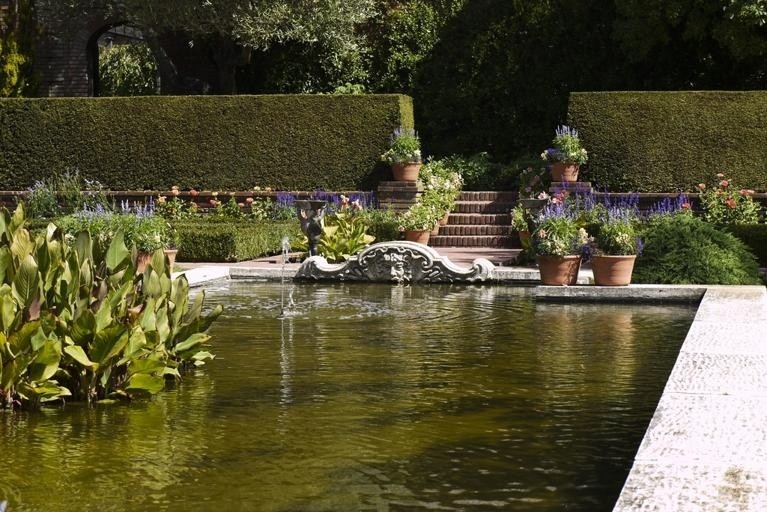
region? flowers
[135,214,177,250]
[540,124,587,162]
[397,151,492,230]
[509,166,688,257]
[378,127,422,162]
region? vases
[537,254,636,287]
[551,162,580,182]
[136,248,178,276]
[392,162,421,181]
[407,225,439,247]
